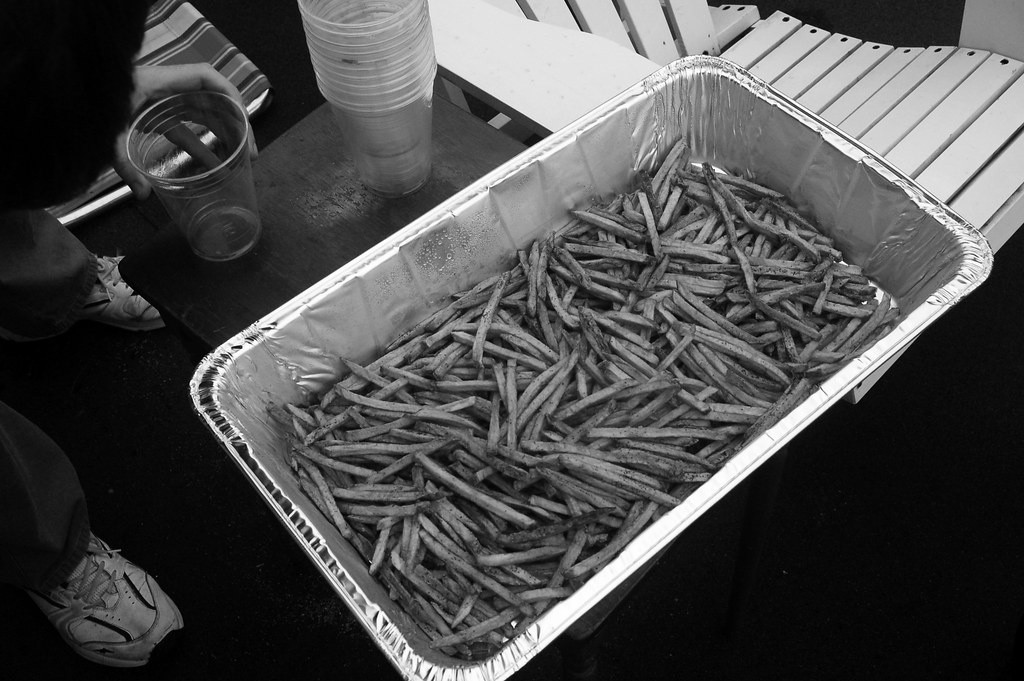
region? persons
[0,1,255,671]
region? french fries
[266,135,902,661]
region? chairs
[363,0,1024,404]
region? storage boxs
[188,56,993,681]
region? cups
[126,89,263,261]
[297,0,438,200]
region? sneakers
[20,526,184,668]
[81,255,165,330]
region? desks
[117,96,532,354]
